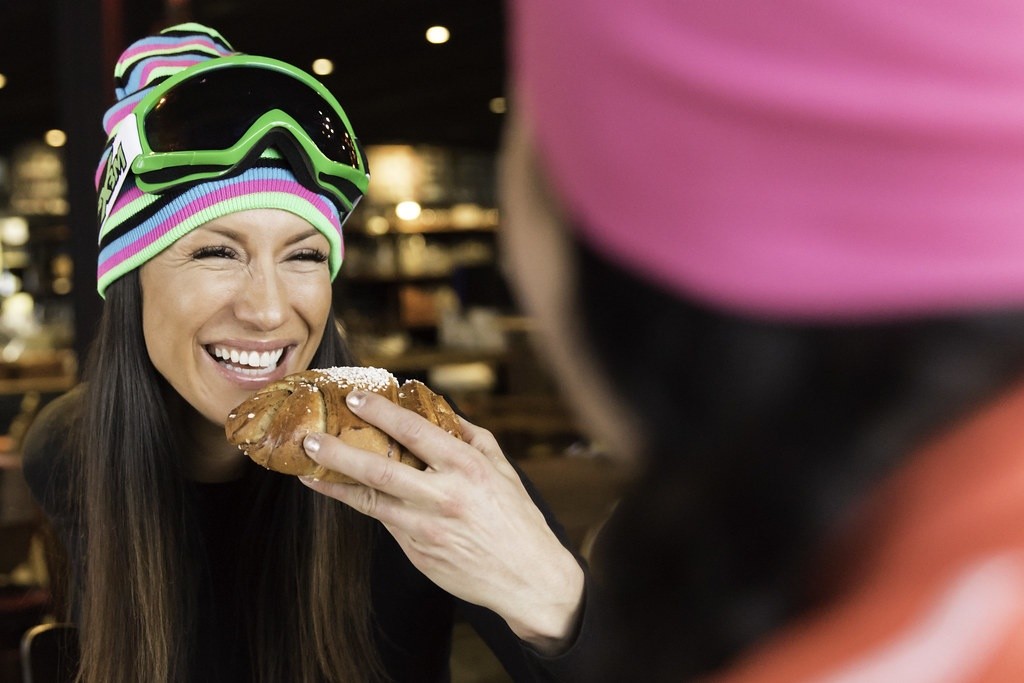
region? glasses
[96,55,370,249]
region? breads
[225,366,464,487]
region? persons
[501,0,1022,683]
[0,21,594,681]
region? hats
[512,0,1024,319]
[95,22,345,300]
